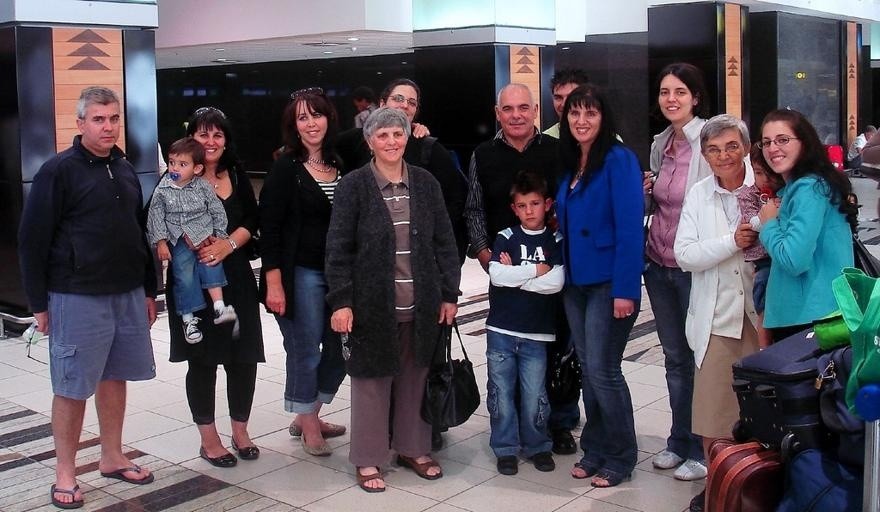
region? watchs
[227,237,237,250]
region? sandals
[572,462,596,479]
[355,466,388,492]
[592,472,622,487]
[399,453,444,480]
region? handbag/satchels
[544,339,582,397]
[853,236,880,277]
[420,359,480,431]
[704,267,879,512]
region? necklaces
[205,173,223,189]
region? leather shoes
[200,443,237,468]
[231,435,259,458]
[301,432,333,456]
[552,430,578,454]
[289,420,346,435]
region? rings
[210,255,214,260]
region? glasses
[757,137,797,151]
[290,88,324,98]
[25,325,49,368]
[193,107,226,121]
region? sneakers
[182,318,204,344]
[214,304,237,324]
[497,456,518,474]
[653,449,683,468]
[673,458,707,482]
[532,451,555,472]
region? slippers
[51,484,84,510]
[101,464,154,485]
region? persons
[143,106,260,468]
[18,86,154,510]
[847,126,879,178]
[465,70,646,487]
[149,136,238,347]
[644,66,860,481]
[261,81,467,495]
